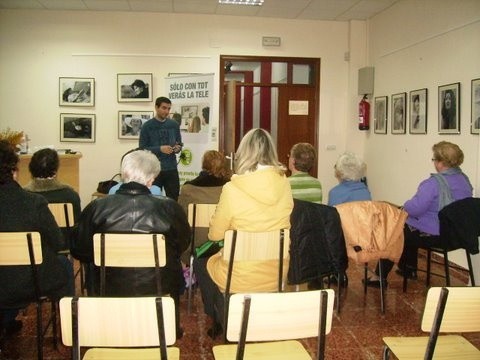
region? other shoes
[0,265,425,342]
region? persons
[69,148,194,341]
[411,95,421,129]
[19,148,83,303]
[176,150,235,294]
[139,97,184,205]
[278,141,324,206]
[201,105,210,124]
[440,88,457,130]
[0,136,63,352]
[362,140,474,289]
[189,116,202,133]
[107,147,163,198]
[320,153,372,289]
[67,88,90,104]
[122,113,145,136]
[196,128,295,338]
[131,79,149,99]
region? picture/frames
[60,111,96,142]
[409,88,427,135]
[373,96,388,134]
[437,82,460,135]
[470,78,480,135]
[117,110,154,140]
[58,76,95,107]
[391,92,407,135]
[117,72,154,103]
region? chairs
[381,286,480,360]
[212,288,336,360]
[44,203,84,297]
[58,296,180,360]
[402,196,480,293]
[326,200,394,312]
[88,232,168,297]
[219,228,292,339]
[186,202,220,320]
[0,232,62,360]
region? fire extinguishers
[358,94,370,131]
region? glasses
[431,158,439,161]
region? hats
[125,114,148,128]
[131,80,145,89]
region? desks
[17,148,84,202]
